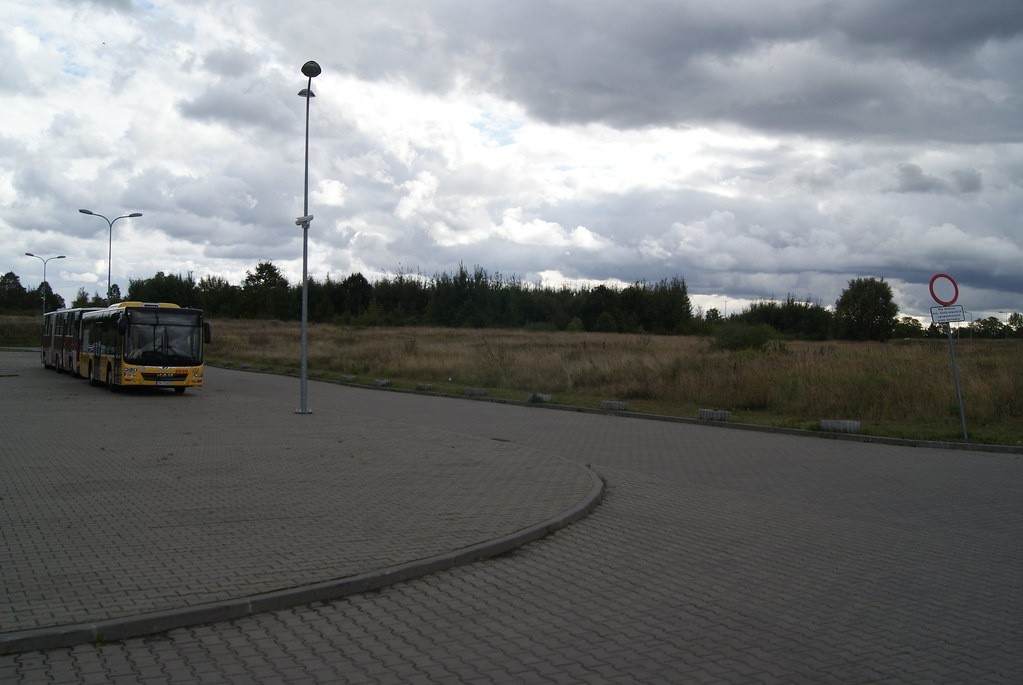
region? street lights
[24,252,66,327]
[295,60,323,414]
[79,209,143,308]
[722,299,729,319]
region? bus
[79,301,212,395]
[42,306,108,377]
